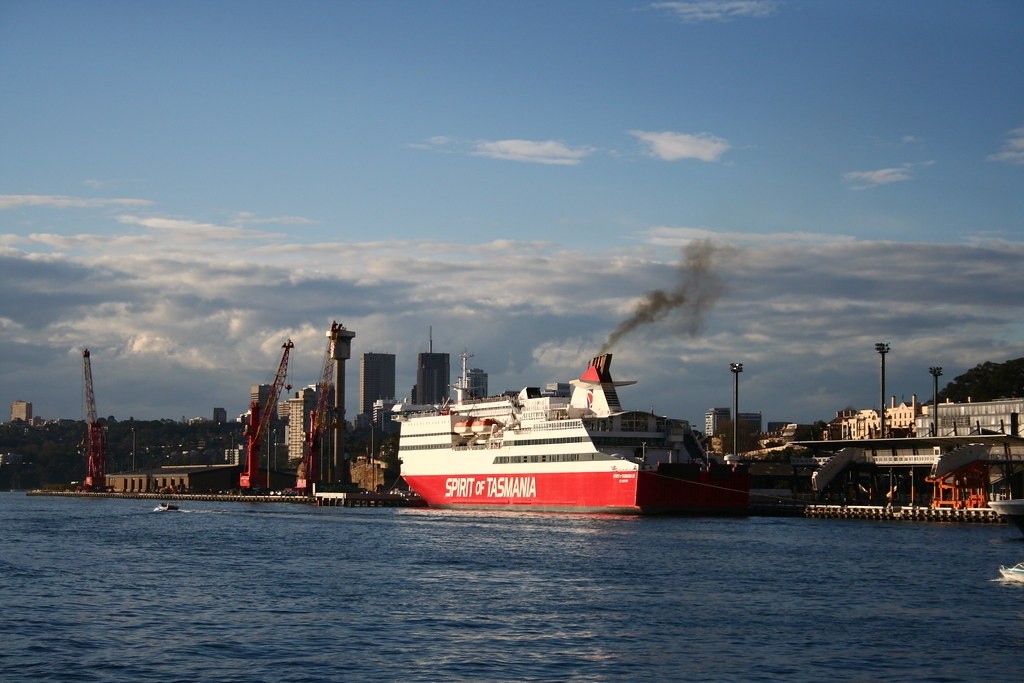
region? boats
[344,492,428,508]
[158,502,179,511]
[1000,563,1024,584]
[397,352,751,517]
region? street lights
[369,420,374,490]
[873,342,887,440]
[929,366,941,436]
[730,361,743,456]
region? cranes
[240,338,295,496]
[81,348,106,492]
[291,322,341,496]
